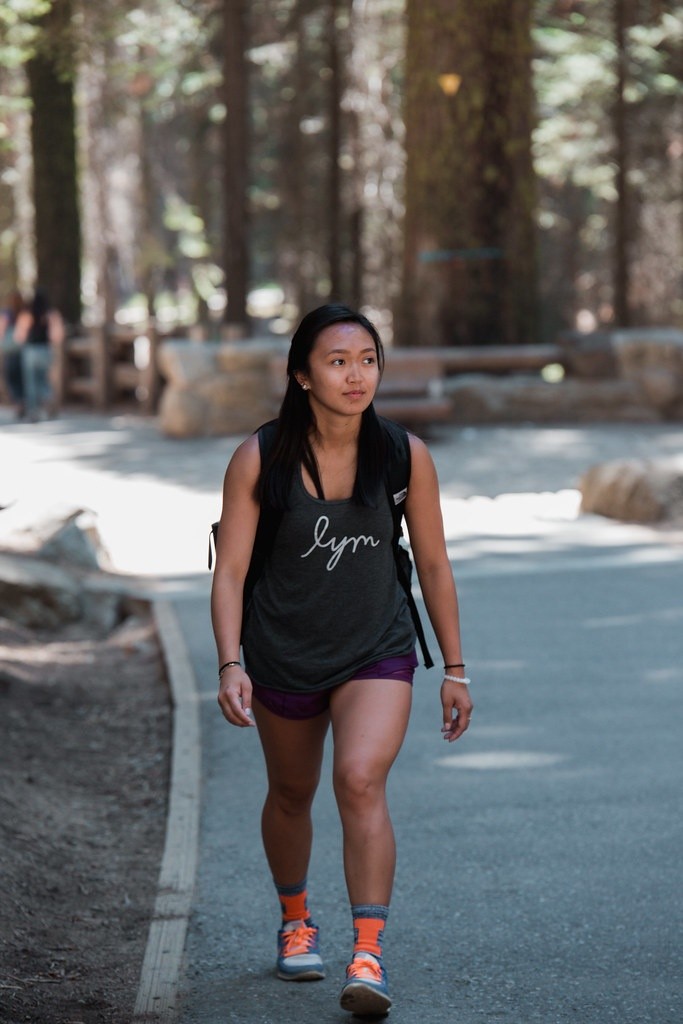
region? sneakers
[277,919,326,980]
[341,951,392,1017]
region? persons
[210,304,473,1019]
[0,285,66,423]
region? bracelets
[444,664,465,669]
[218,661,241,680]
[444,675,470,684]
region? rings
[467,714,472,720]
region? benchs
[371,398,456,440]
